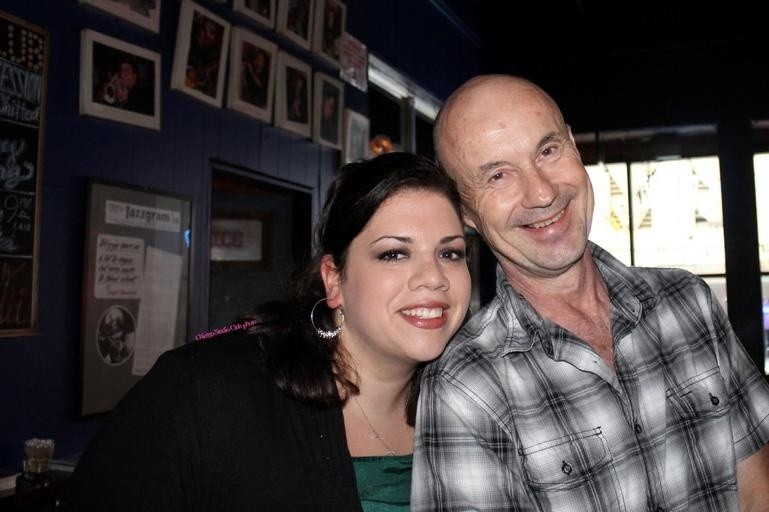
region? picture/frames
[79,176,196,417]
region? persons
[103,60,149,114]
[186,20,224,97]
[410,73,769,512]
[98,309,135,364]
[241,52,268,108]
[57,152,472,512]
[322,93,338,144]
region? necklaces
[351,392,396,456]
